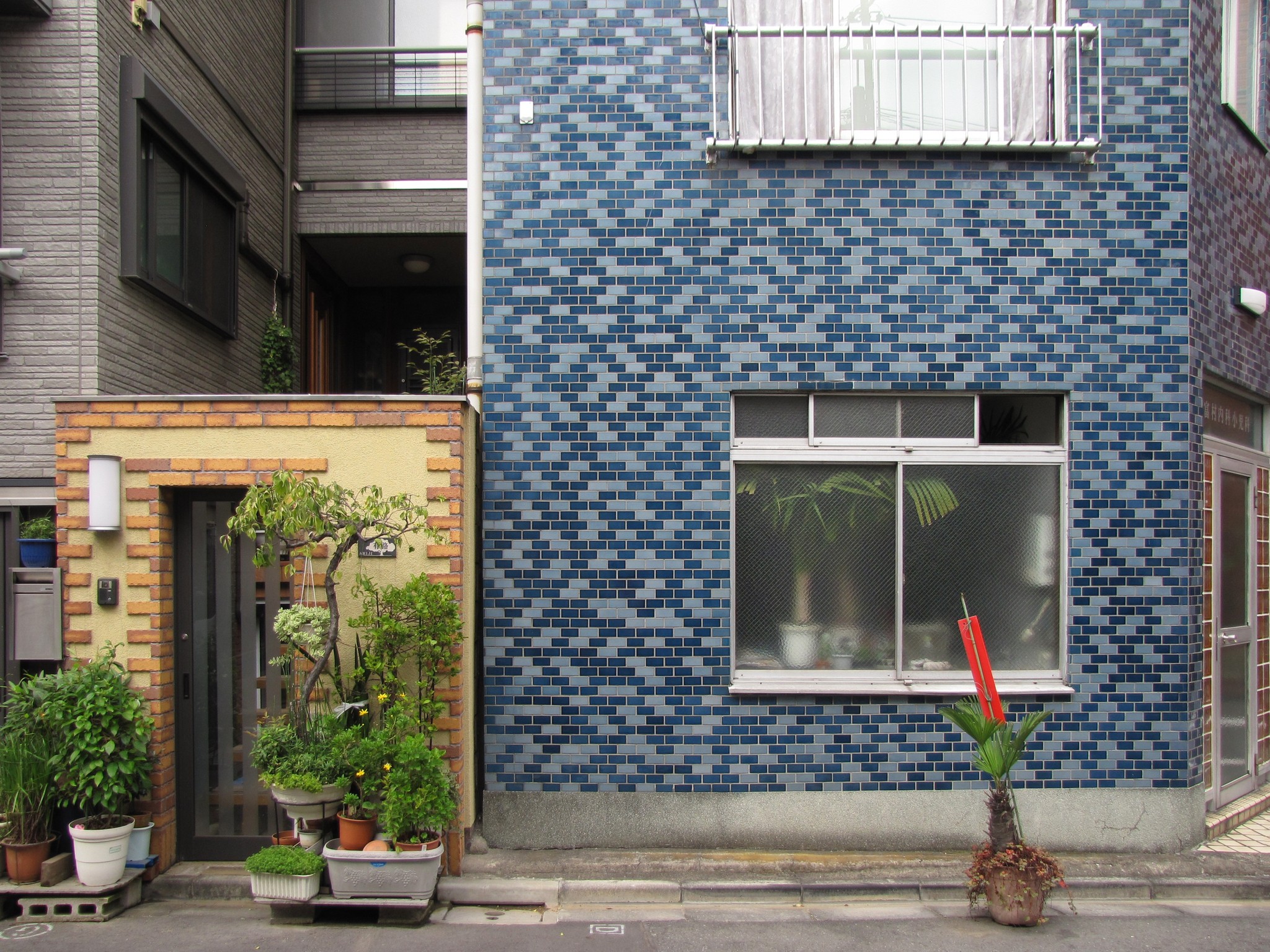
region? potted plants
[17,517,56,568]
[937,694,1081,927]
[219,471,468,901]
[0,640,155,886]
[736,463,960,670]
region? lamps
[1233,285,1266,318]
